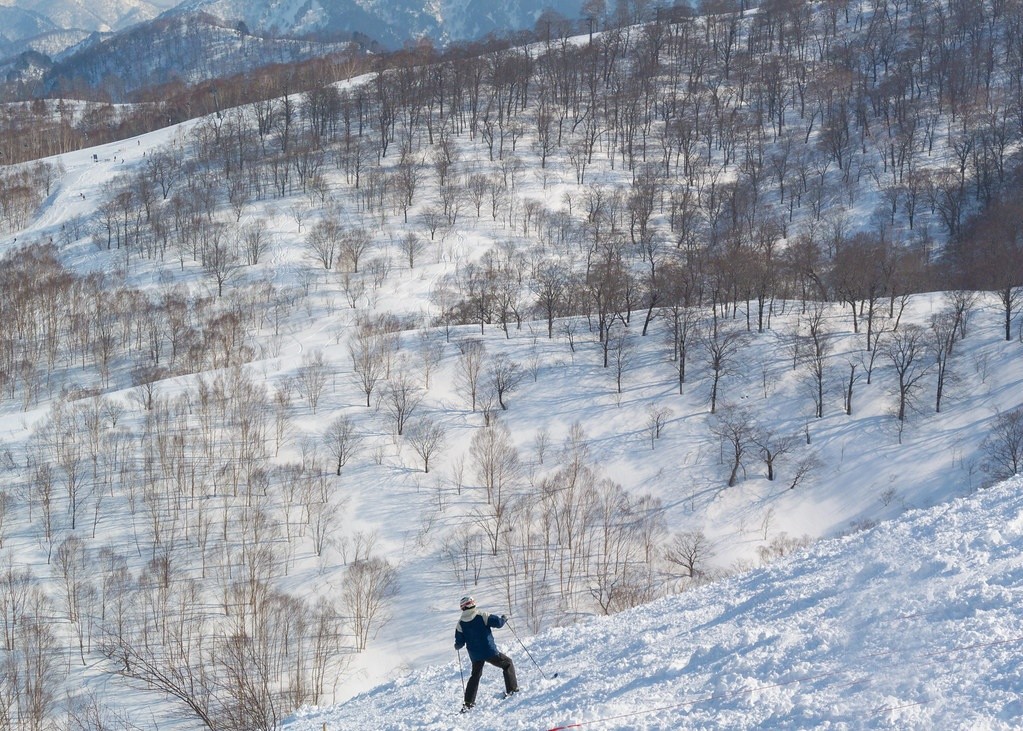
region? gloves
[501,614,509,623]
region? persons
[454,596,519,711]
[11,139,177,244]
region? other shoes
[508,688,521,694]
[466,702,476,710]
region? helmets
[460,596,475,612]
